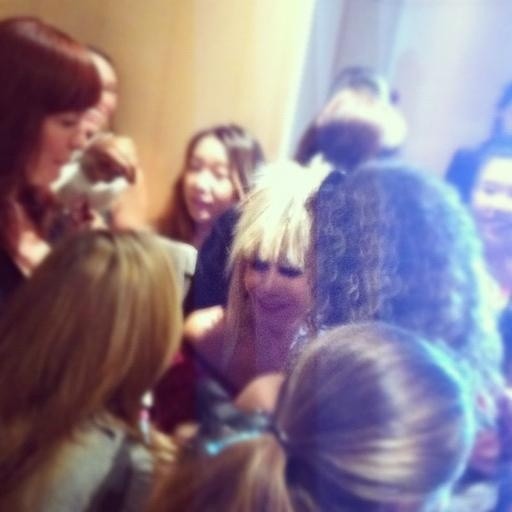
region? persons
[0,229,188,512]
[179,157,349,395]
[441,82,510,380]
[181,87,412,316]
[0,13,101,301]
[442,132,511,512]
[45,38,152,231]
[145,118,268,251]
[325,62,398,107]
[306,155,506,441]
[144,314,478,511]
